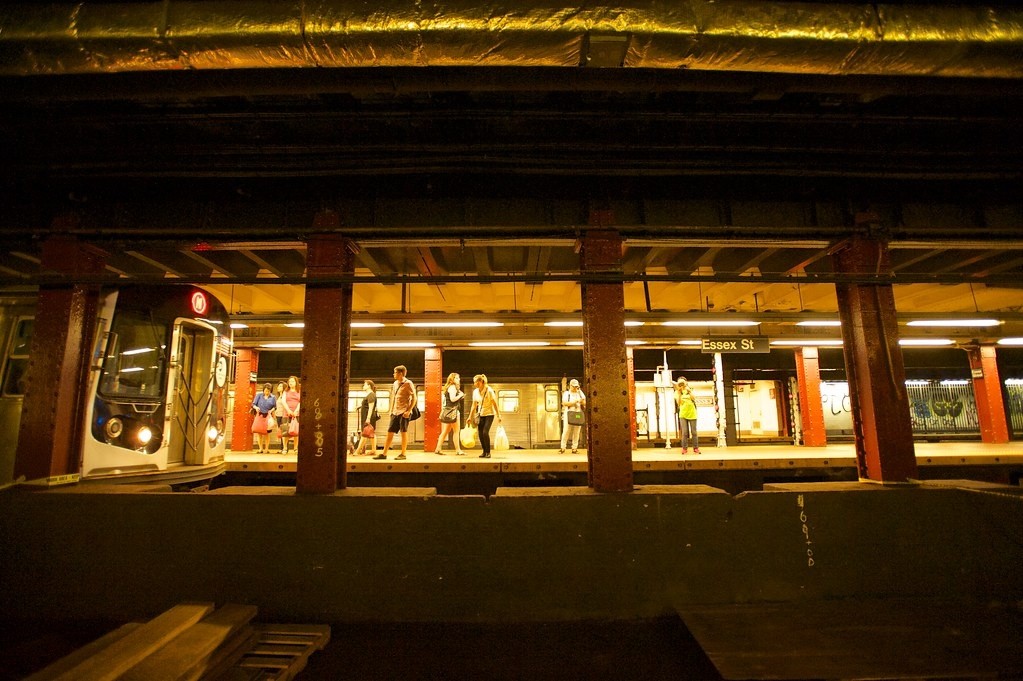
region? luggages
[350,409,366,454]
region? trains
[0,284,236,490]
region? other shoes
[435,449,446,455]
[572,449,580,454]
[456,450,468,456]
[682,447,687,454]
[558,449,566,455]
[373,454,386,459]
[353,452,360,456]
[694,447,702,454]
[365,452,376,456]
[395,454,406,460]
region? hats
[570,378,579,387]
[677,379,686,387]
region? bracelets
[584,406,585,408]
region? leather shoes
[480,453,490,458]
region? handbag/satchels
[568,411,585,426]
[494,424,509,452]
[361,423,375,438]
[278,416,299,437]
[266,410,278,430]
[410,405,421,420]
[439,406,457,423]
[471,413,480,428]
[249,408,256,416]
[366,411,380,423]
[460,423,478,448]
[251,412,268,435]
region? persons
[282,376,300,455]
[435,373,467,455]
[673,377,701,454]
[373,365,416,460]
[467,374,502,458]
[249,383,277,454]
[276,381,288,455]
[353,380,377,456]
[558,379,586,454]
